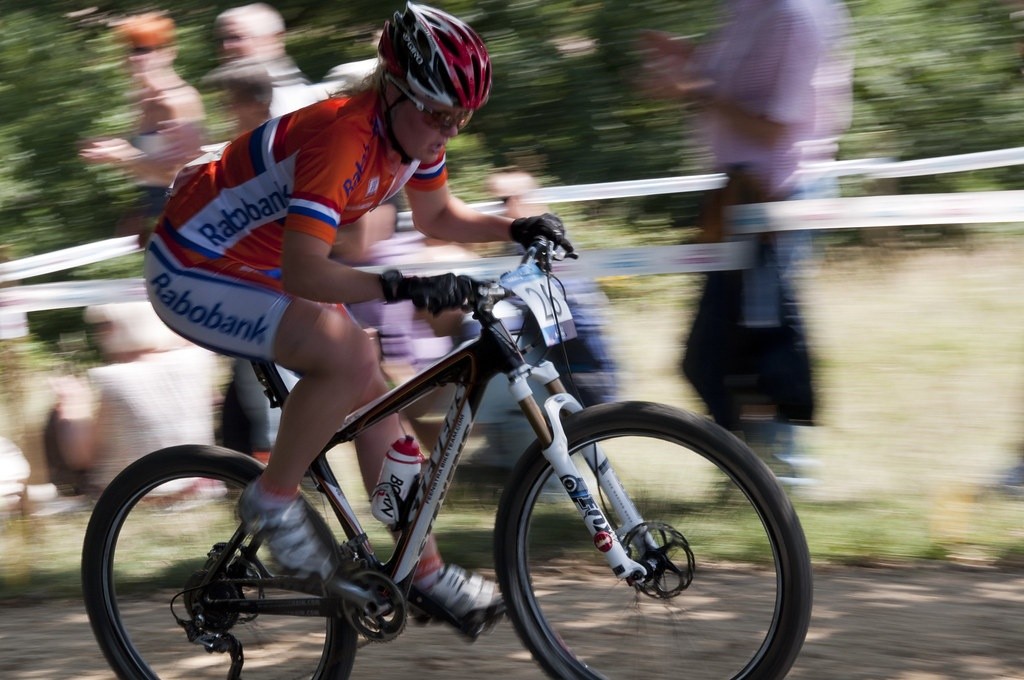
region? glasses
[379,70,475,130]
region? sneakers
[398,559,507,624]
[237,480,318,573]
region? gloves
[509,212,575,261]
[378,266,469,319]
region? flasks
[371,433,420,528]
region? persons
[136,0,572,627]
[637,0,860,502]
[2,3,621,516]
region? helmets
[374,4,496,113]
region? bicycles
[81,211,815,679]
